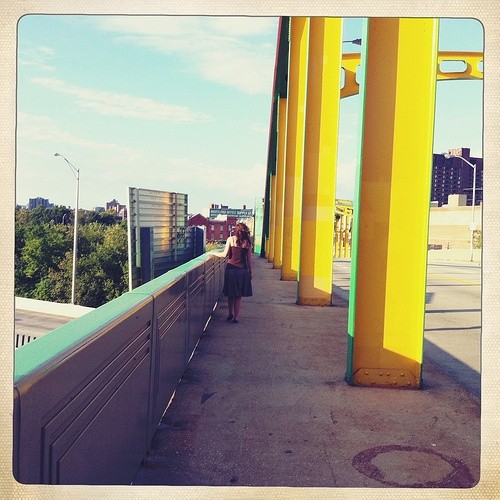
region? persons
[206,222,253,324]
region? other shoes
[232,320,239,323]
[226,314,233,321]
